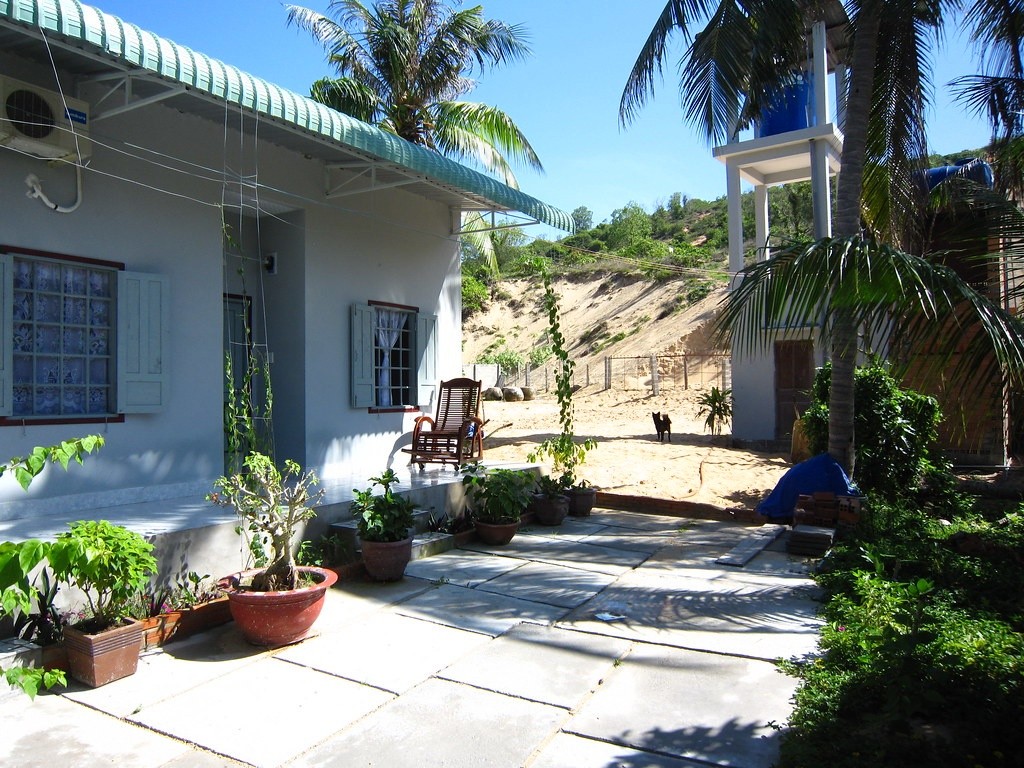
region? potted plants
[207,454,339,649]
[52,518,156,688]
[459,462,536,548]
[564,471,598,518]
[353,476,418,583]
[534,475,570,527]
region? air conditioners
[0,74,90,164]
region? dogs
[652,412,672,442]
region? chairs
[401,378,484,470]
